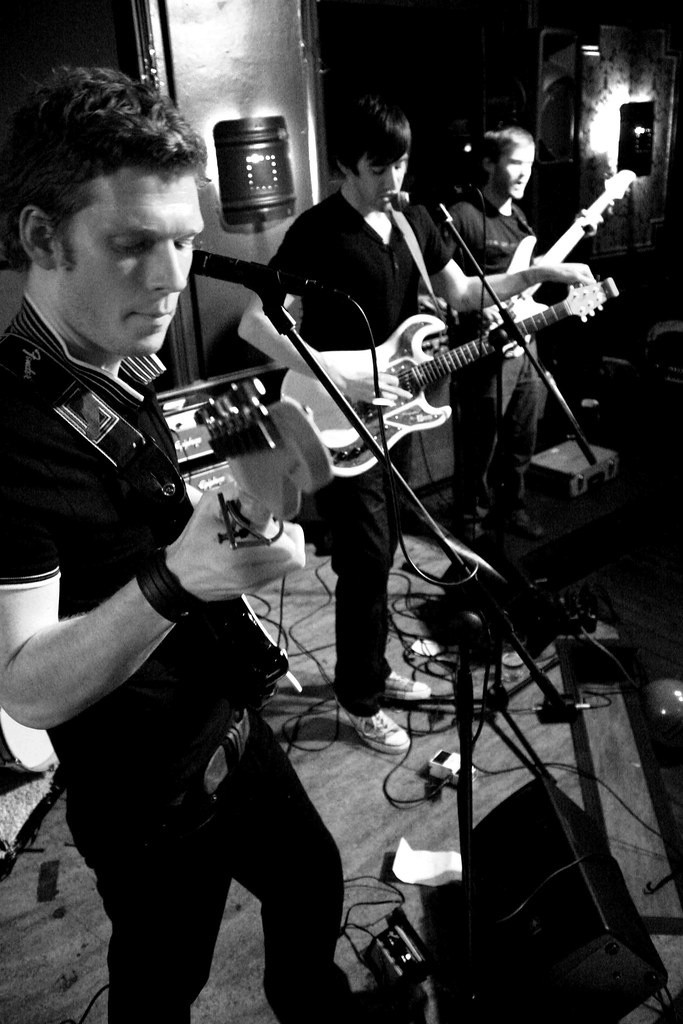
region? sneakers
[383,670,431,701]
[334,694,411,754]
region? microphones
[188,249,351,302]
[391,183,476,210]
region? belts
[170,706,250,806]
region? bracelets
[133,547,208,624]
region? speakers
[0,0,144,271]
[467,773,669,1024]
[507,26,579,166]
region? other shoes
[505,510,545,539]
[352,986,428,1024]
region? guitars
[193,376,333,538]
[279,272,621,479]
[484,170,638,359]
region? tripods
[382,207,599,783]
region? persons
[1,65,359,1024]
[238,97,599,755]
[440,124,565,542]
